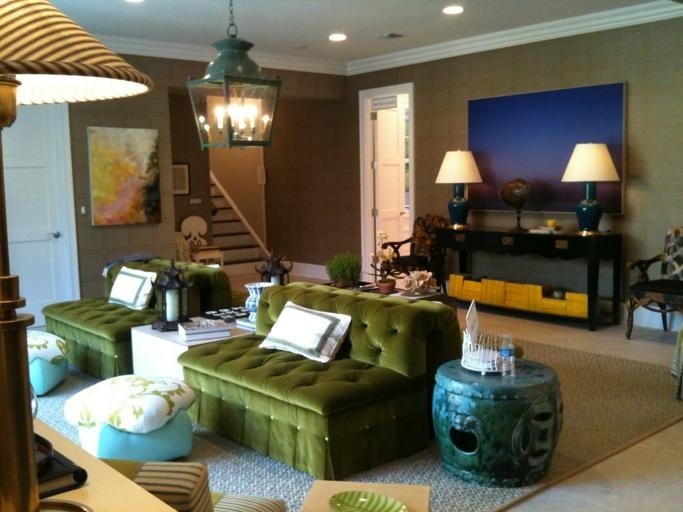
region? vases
[377,279,395,294]
[241,281,275,321]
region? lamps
[148,260,190,331]
[186,0,283,152]
[0,0,152,512]
[252,248,294,288]
[434,149,482,226]
[560,141,621,236]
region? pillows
[104,265,157,312]
[254,302,351,363]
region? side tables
[131,322,252,379]
[434,355,561,488]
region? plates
[328,489,407,511]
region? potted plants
[327,251,363,287]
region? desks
[435,224,621,334]
[321,281,457,318]
[298,479,429,512]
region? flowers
[369,247,405,281]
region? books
[34,448,86,499]
[177,318,231,342]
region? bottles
[499,334,517,379]
[243,281,275,322]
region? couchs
[26,328,66,397]
[40,257,252,380]
[62,373,194,460]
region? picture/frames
[169,161,192,196]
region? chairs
[181,214,224,266]
[98,459,289,512]
[625,229,682,337]
[384,216,448,287]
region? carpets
[0,298,682,512]
